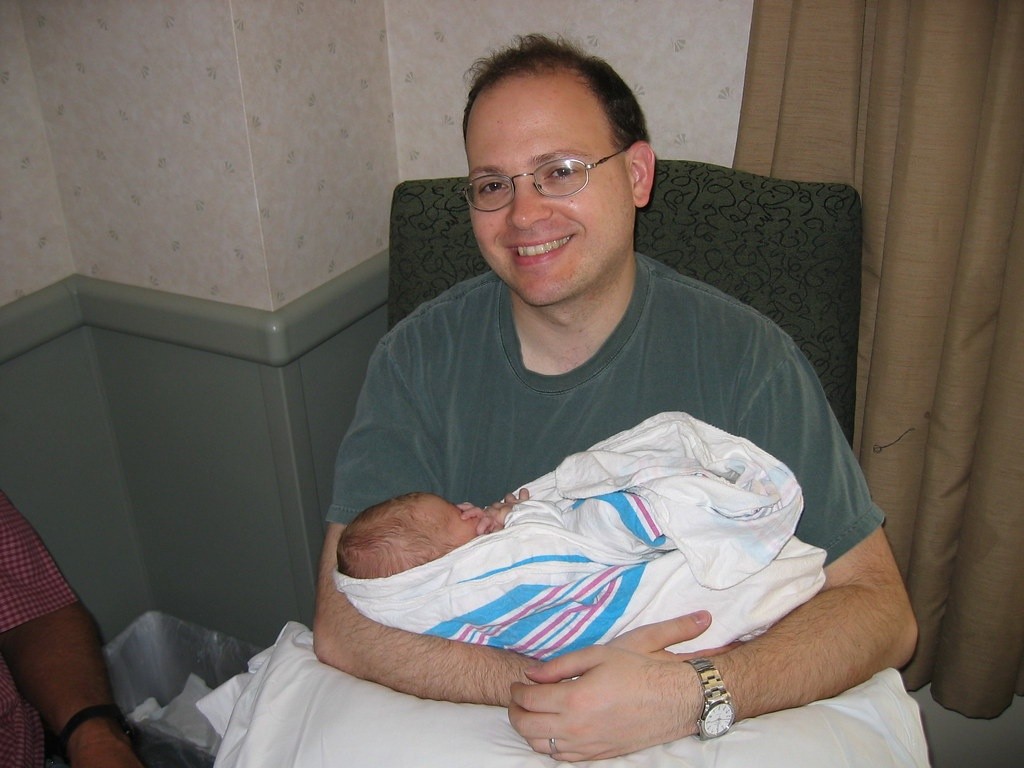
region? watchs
[684,653,733,742]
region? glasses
[459,145,629,212]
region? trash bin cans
[101,609,266,768]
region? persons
[307,37,923,767]
[1,491,141,766]
[334,489,537,580]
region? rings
[548,738,559,755]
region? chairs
[387,159,861,450]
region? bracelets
[59,704,136,760]
